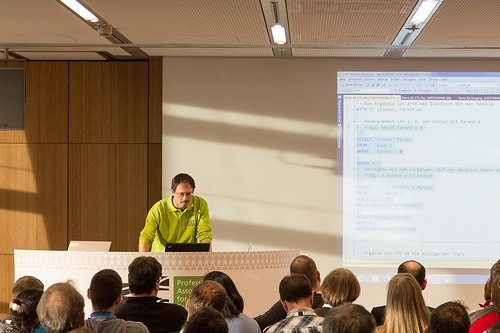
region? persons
[139,173,212,252]
[0,255,500,333]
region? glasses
[120,294,127,301]
[175,192,193,197]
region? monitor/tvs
[165,243,210,252]
